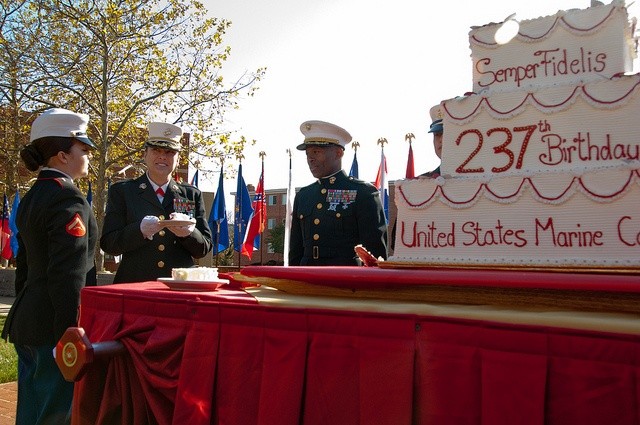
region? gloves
[139,215,165,240]
[167,218,197,238]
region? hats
[30,107,98,150]
[427,104,443,134]
[296,120,352,151]
[146,122,184,152]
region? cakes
[169,211,189,221]
[387,0,640,269]
[171,267,217,281]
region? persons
[418,103,444,177]
[0,108,99,424]
[99,121,213,284]
[286,120,388,267]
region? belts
[303,246,338,260]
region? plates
[160,219,195,228]
[156,275,229,292]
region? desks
[72,281,640,425]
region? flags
[102,174,119,273]
[85,181,93,215]
[241,170,267,261]
[190,172,202,188]
[373,151,389,227]
[1,191,11,267]
[172,170,183,182]
[402,145,418,180]
[349,153,360,178]
[231,167,255,251]
[208,168,229,257]
[284,166,294,268]
[7,187,21,262]
